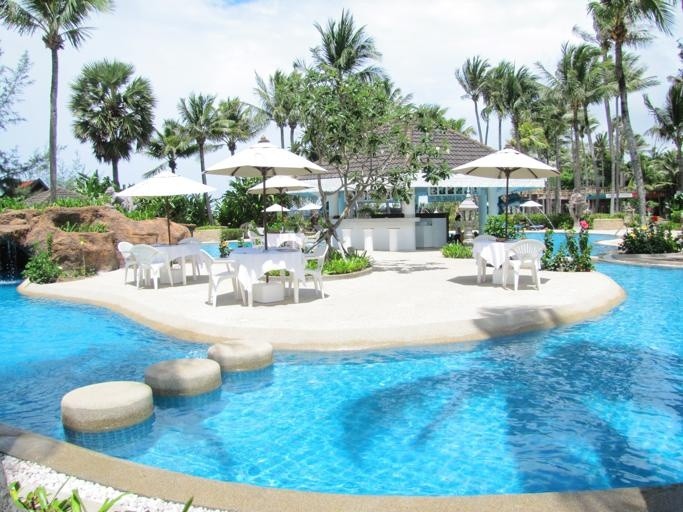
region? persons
[453,211,462,235]
[309,210,320,232]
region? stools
[362,229,375,252]
[340,228,353,250]
[387,228,399,251]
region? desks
[471,240,546,290]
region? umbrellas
[449,143,561,240]
[297,201,322,210]
[113,168,218,268]
[519,199,544,214]
[260,204,289,213]
[248,175,323,230]
[201,132,328,284]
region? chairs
[502,238,547,291]
[471,233,500,285]
[116,224,332,308]
